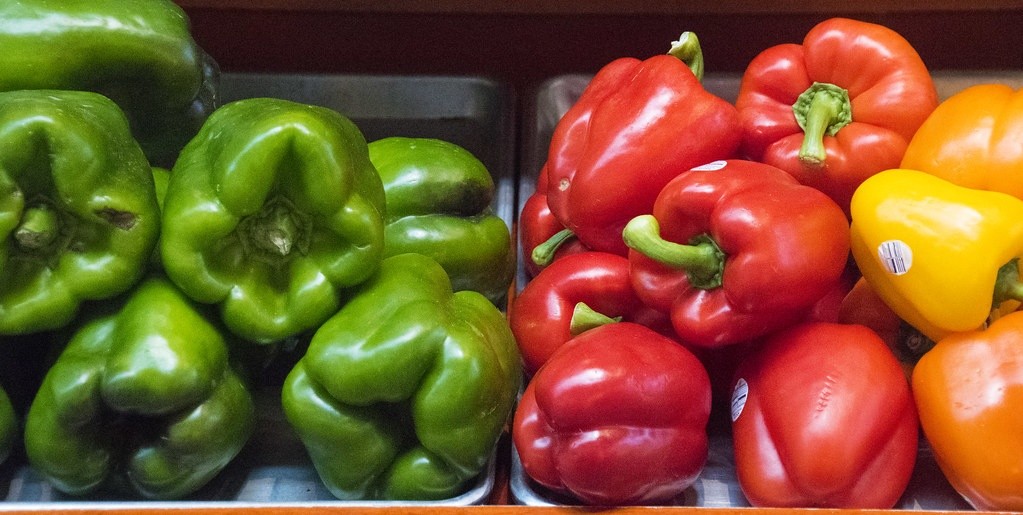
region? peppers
[0,0,1023,510]
